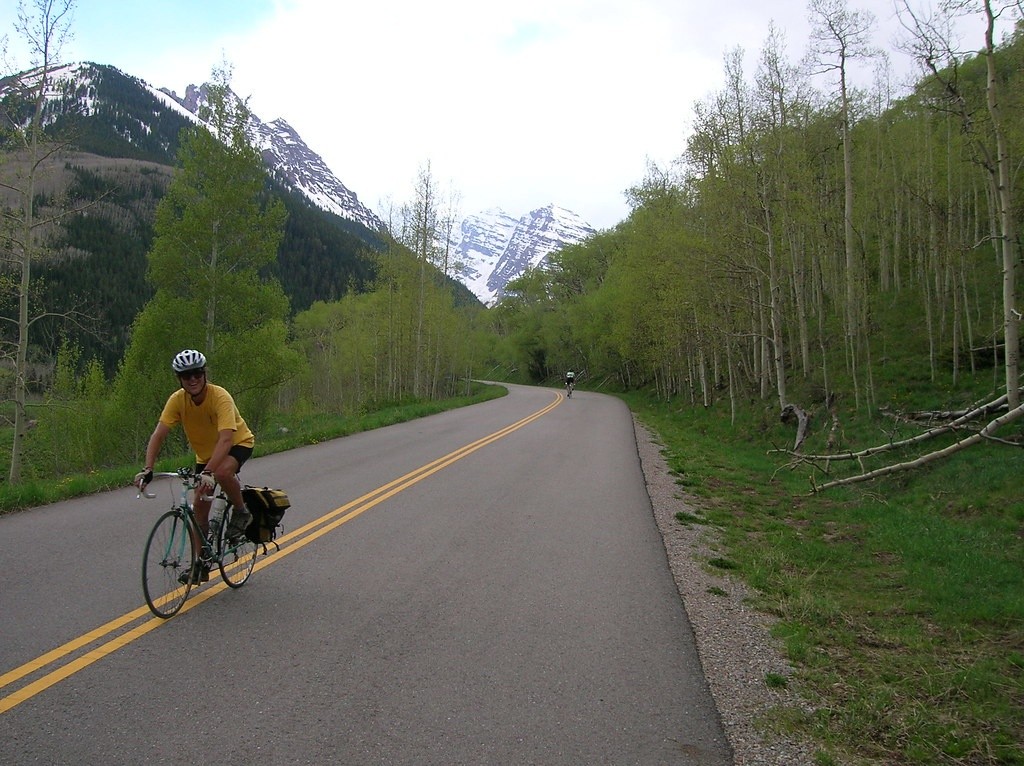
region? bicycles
[565,383,573,399]
[136,467,259,620]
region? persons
[566,368,575,397]
[133,350,254,583]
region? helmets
[172,350,208,372]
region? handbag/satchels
[242,485,291,555]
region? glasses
[179,369,204,379]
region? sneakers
[224,509,254,540]
[182,564,210,583]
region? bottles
[209,491,226,527]
